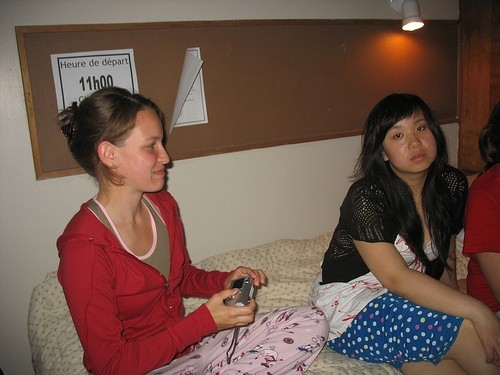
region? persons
[309,92,500,375]
[462,102,500,323]
[57,87,329,375]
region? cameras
[225,276,254,307]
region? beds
[27,228,471,375]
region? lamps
[390,0,424,31]
